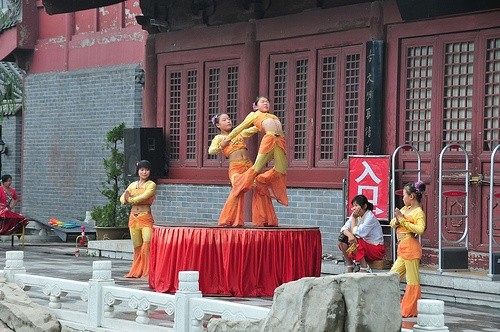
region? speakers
[123,126,164,180]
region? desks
[148,222,322,297]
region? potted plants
[90,122,131,240]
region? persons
[209,113,260,227]
[120,159,157,279]
[337,195,385,273]
[0,175,28,235]
[389,181,426,317]
[220,96,289,207]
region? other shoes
[360,264,373,273]
[347,262,356,269]
